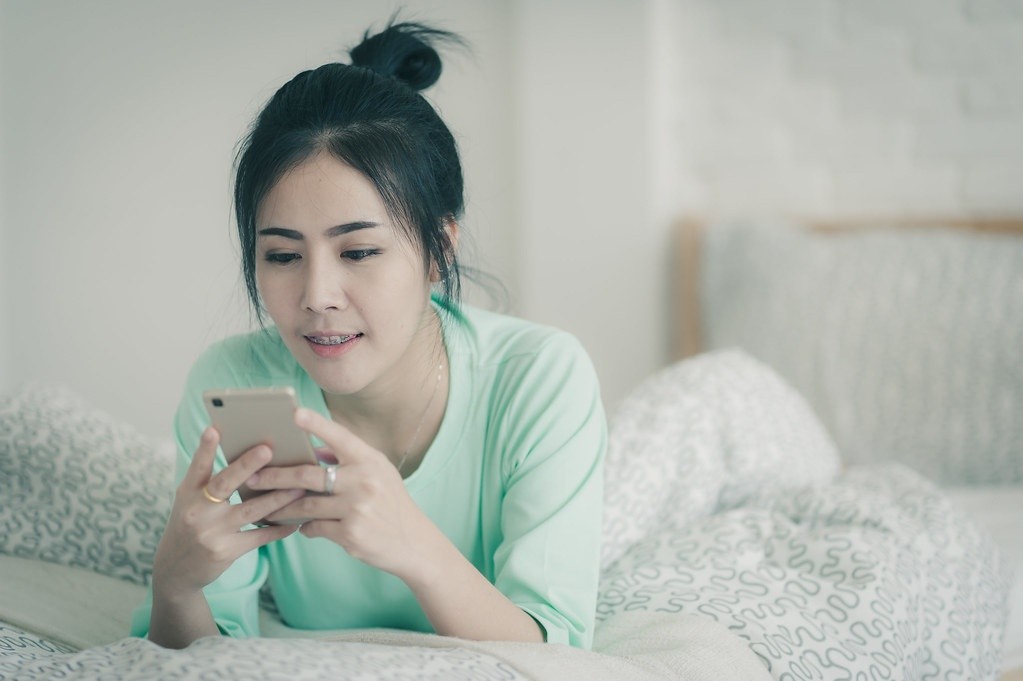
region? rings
[202,485,227,504]
[324,465,336,496]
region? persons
[127,7,610,650]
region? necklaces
[396,358,446,470]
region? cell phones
[203,386,322,527]
